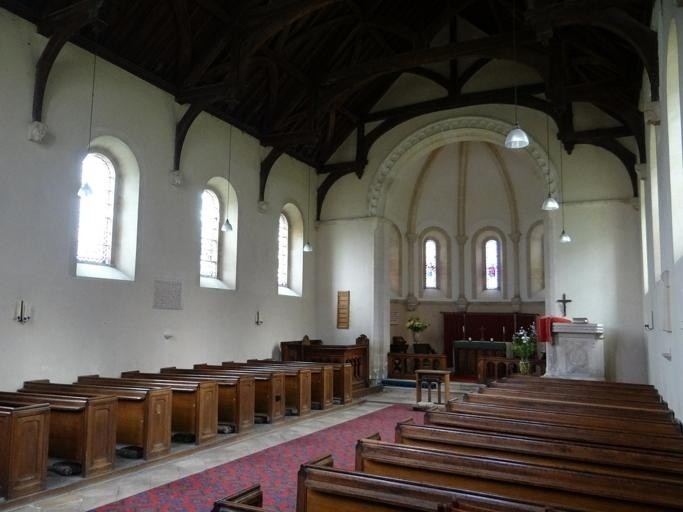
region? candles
[502,326,505,333]
[462,326,465,332]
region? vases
[414,332,423,344]
[519,361,530,375]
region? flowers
[510,321,537,360]
[405,316,431,331]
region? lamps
[558,139,571,243]
[541,115,559,210]
[303,166,312,251]
[76,56,96,198]
[504,0,529,149]
[221,118,233,232]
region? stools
[415,369,449,402]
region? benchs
[0,358,352,509]
[211,373,683,512]
